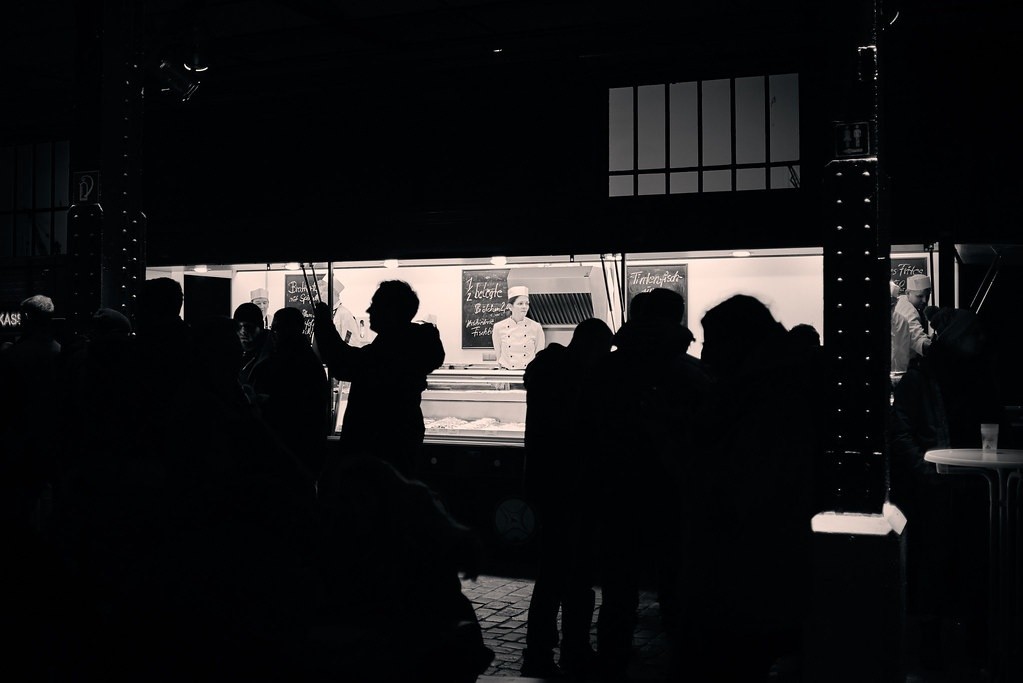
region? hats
[507,286,529,299]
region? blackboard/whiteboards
[890,257,929,305]
[462,268,512,348]
[625,263,689,328]
[284,274,335,336]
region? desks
[924,449,1023,683]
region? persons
[491,285,545,370]
[519,289,1023,683]
[891,274,933,372]
[0,274,480,683]
